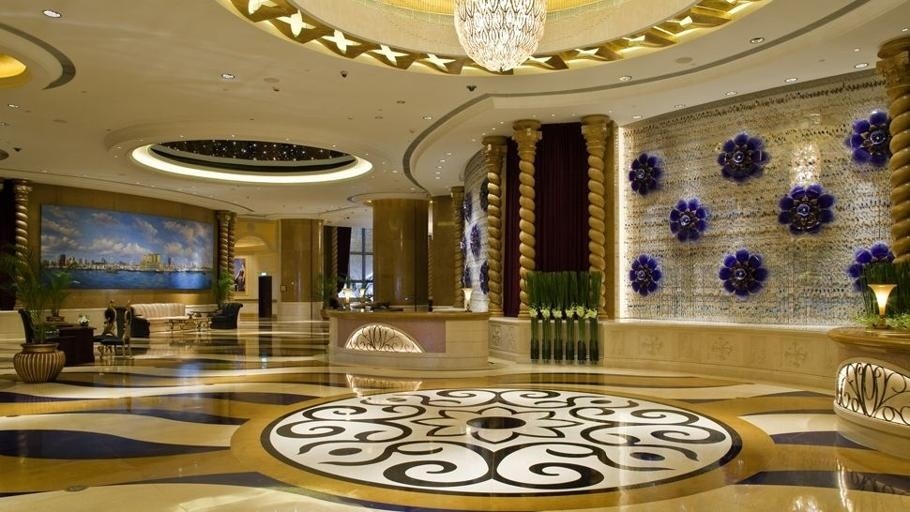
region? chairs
[19,306,133,362]
[209,302,243,328]
[129,307,149,337]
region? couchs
[131,303,190,331]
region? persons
[238,270,244,291]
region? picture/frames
[234,255,248,296]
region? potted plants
[39,261,74,322]
[0,236,76,382]
[312,274,340,320]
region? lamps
[342,288,354,311]
[867,283,899,328]
[453,0,546,72]
